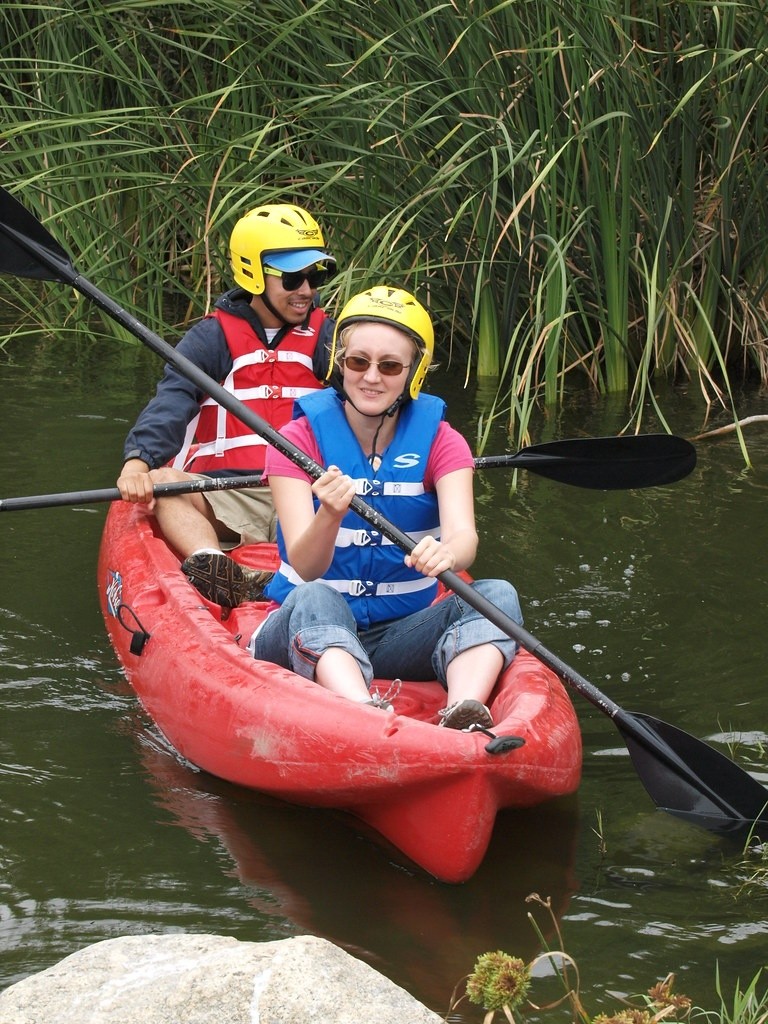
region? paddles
[0,186,768,840]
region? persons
[253,284,523,730]
[119,203,339,605]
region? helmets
[325,285,434,401]
[230,203,325,297]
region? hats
[262,250,336,274]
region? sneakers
[180,548,276,608]
[372,693,495,731]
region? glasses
[263,264,330,292]
[342,355,412,376]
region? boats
[93,463,583,879]
